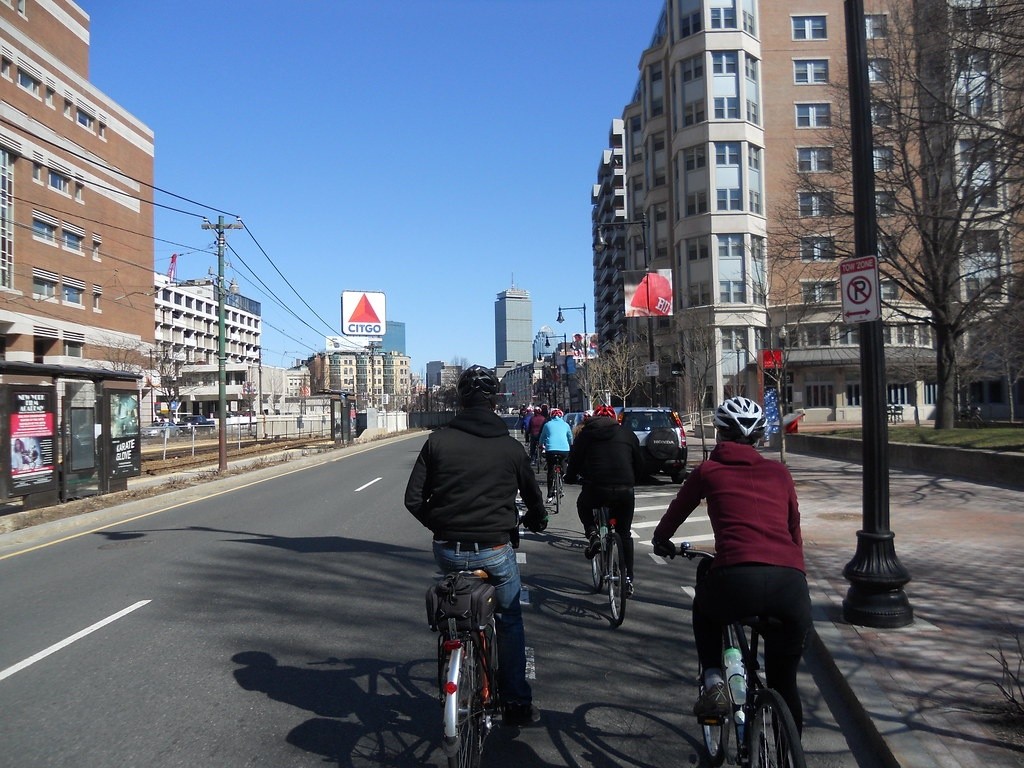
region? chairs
[886,406,905,423]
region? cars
[140,422,181,439]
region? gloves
[563,475,577,484]
[652,539,676,560]
[523,512,548,533]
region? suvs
[613,406,688,485]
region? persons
[153,418,158,422]
[572,334,598,357]
[627,270,671,317]
[520,404,574,503]
[404,364,549,727]
[160,418,165,422]
[572,404,644,599]
[652,393,812,768]
[12,438,38,471]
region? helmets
[593,404,617,421]
[713,396,767,442]
[549,408,564,417]
[455,365,501,406]
[522,403,548,414]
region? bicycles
[524,426,543,475]
[424,514,546,768]
[653,542,807,768]
[562,477,629,627]
[543,446,571,513]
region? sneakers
[501,699,541,725]
[620,582,632,601]
[585,535,601,559]
[692,679,732,716]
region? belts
[596,484,634,491]
[441,539,495,550]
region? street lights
[592,209,658,408]
[556,303,590,411]
[544,332,573,412]
[538,349,557,408]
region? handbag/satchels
[426,572,496,631]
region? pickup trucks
[174,415,216,435]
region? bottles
[600,524,608,551]
[724,647,748,705]
